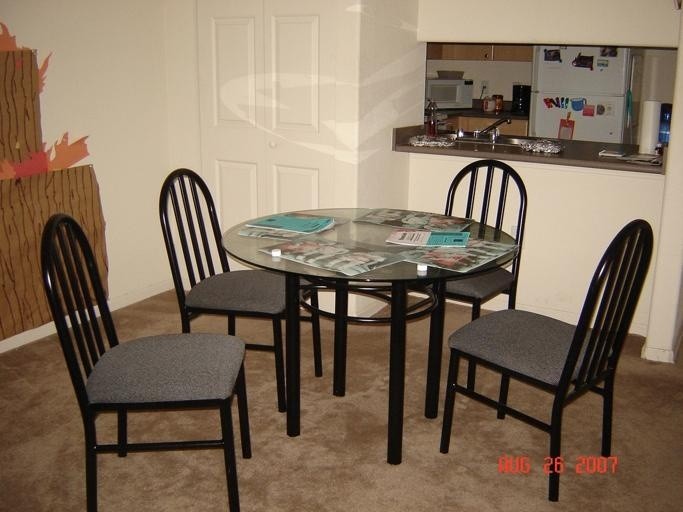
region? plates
[437,70,464,80]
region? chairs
[41,214,251,512]
[407,158,527,419]
[159,168,322,412]
[439,220,654,502]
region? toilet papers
[638,100,661,156]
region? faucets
[480,116,512,136]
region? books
[246,211,336,235]
[387,228,473,249]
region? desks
[220,208,519,464]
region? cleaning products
[426,98,438,139]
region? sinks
[455,135,539,148]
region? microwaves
[425,78,473,109]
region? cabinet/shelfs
[427,43,532,61]
[446,116,528,136]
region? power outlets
[481,81,491,94]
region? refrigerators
[528,45,633,146]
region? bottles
[479,84,503,113]
[659,112,670,142]
[427,99,439,138]
[655,143,662,155]
